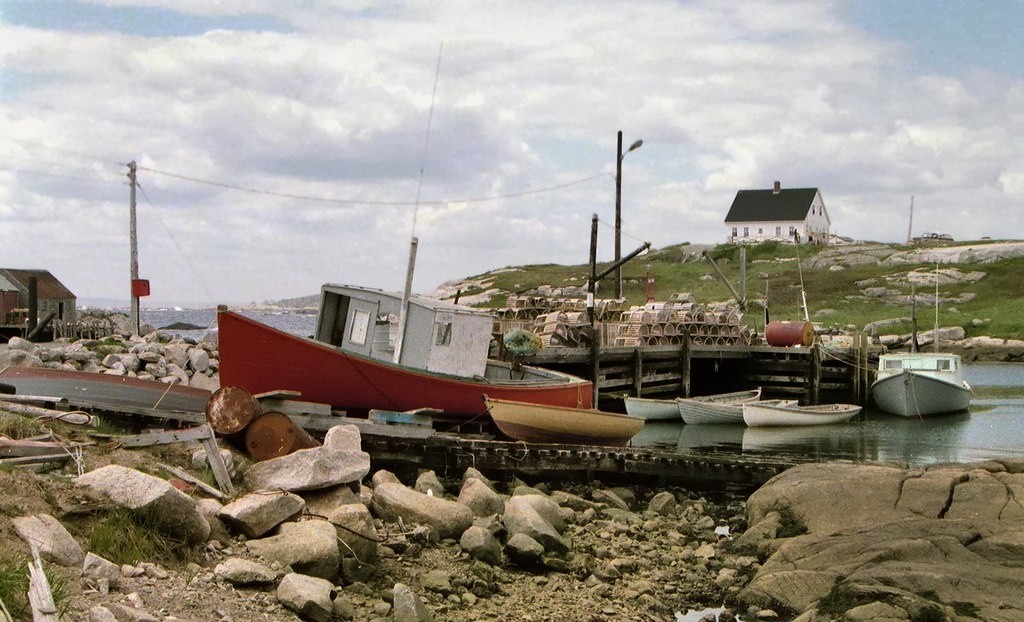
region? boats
[741,402,864,428]
[214,279,595,437]
[622,385,764,422]
[481,392,646,448]
[871,262,975,419]
[674,395,800,425]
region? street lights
[613,130,644,299]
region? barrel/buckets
[766,321,814,347]
[205,385,270,441]
[244,410,322,462]
